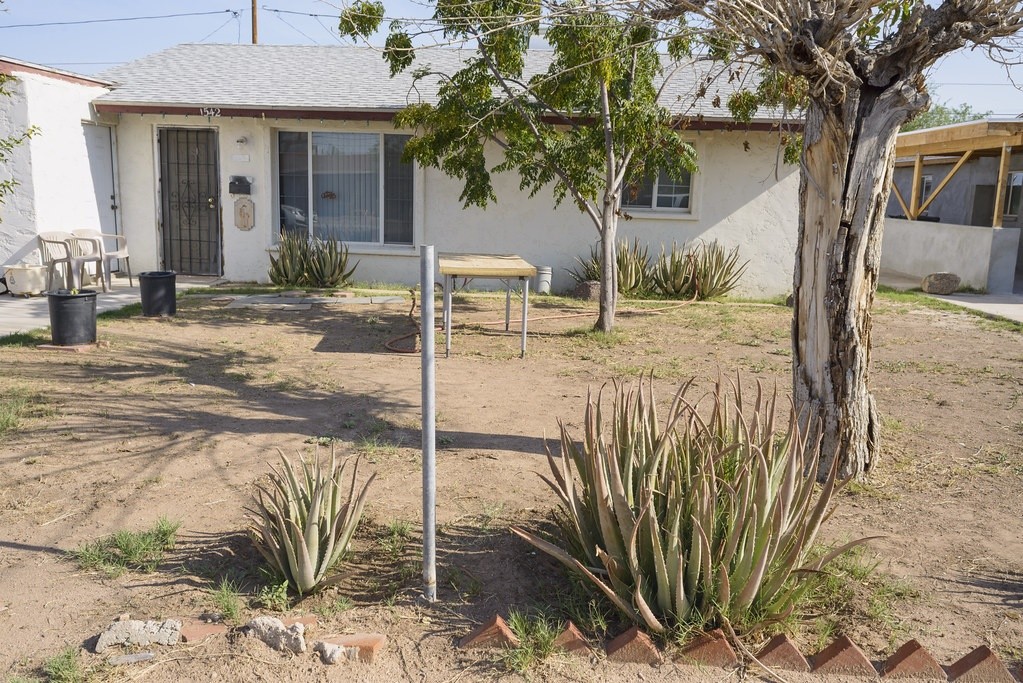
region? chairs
[40,231,103,292]
[72,229,133,291]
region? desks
[438,253,537,358]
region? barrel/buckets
[533,266,552,294]
[137,271,176,317]
[43,289,102,346]
[1,265,48,298]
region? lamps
[235,137,247,149]
